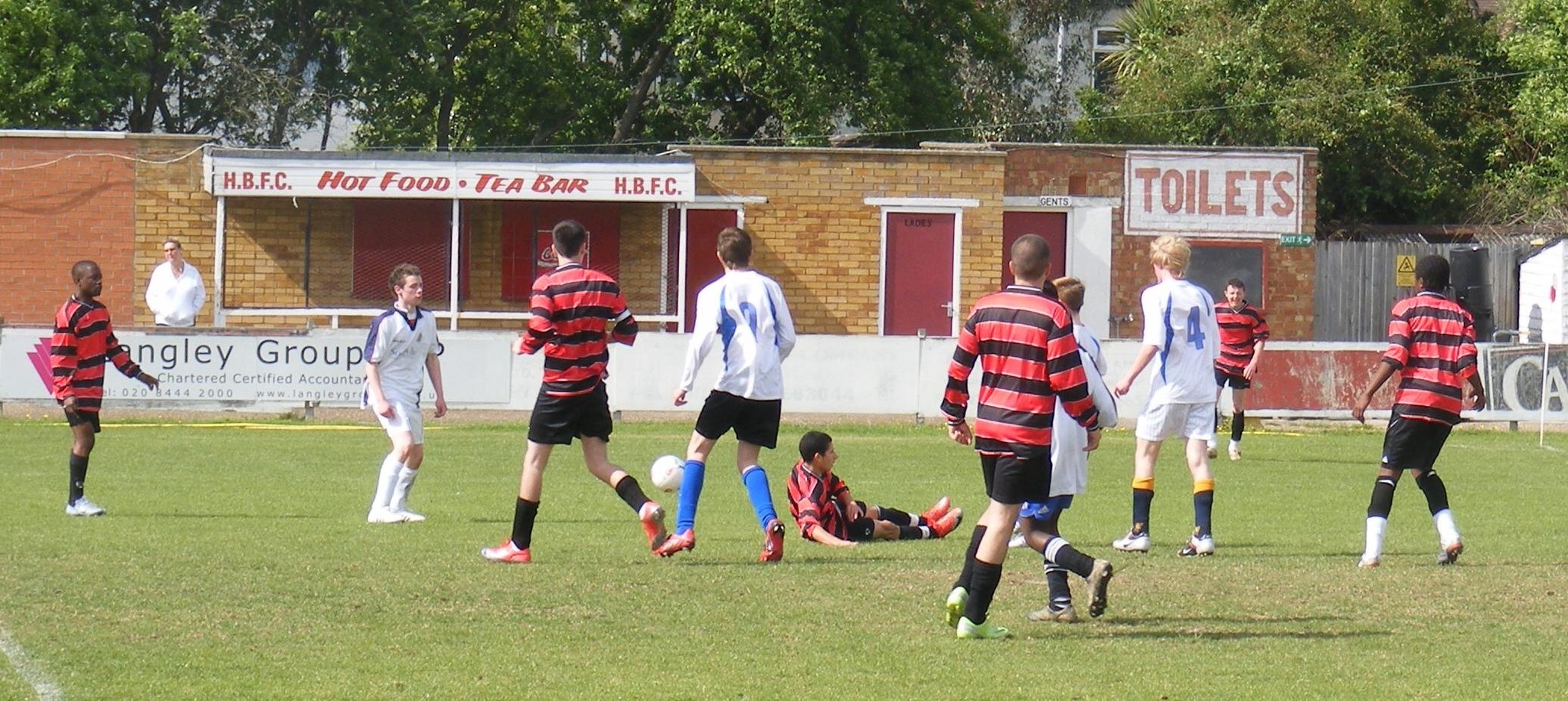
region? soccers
[650,455,686,492]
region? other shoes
[1012,521,1021,534]
[1008,533,1027,547]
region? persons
[1113,235,1222,557]
[940,235,1102,641]
[652,226,795,562]
[145,238,206,328]
[481,221,666,563]
[1008,276,1113,621]
[51,261,160,516]
[1204,278,1270,460]
[788,432,962,547]
[1354,254,1486,567]
[360,264,447,523]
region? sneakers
[957,612,1009,640]
[1359,553,1380,569]
[652,528,695,558]
[1113,528,1151,552]
[760,519,785,562]
[368,508,410,523]
[1177,530,1214,557]
[638,501,667,551]
[1228,447,1241,460]
[1083,559,1114,617]
[945,586,970,628]
[66,497,105,516]
[1027,605,1078,623]
[482,537,531,563]
[921,496,950,526]
[396,510,426,522]
[1437,536,1464,565]
[1207,447,1217,459]
[933,508,963,538]
